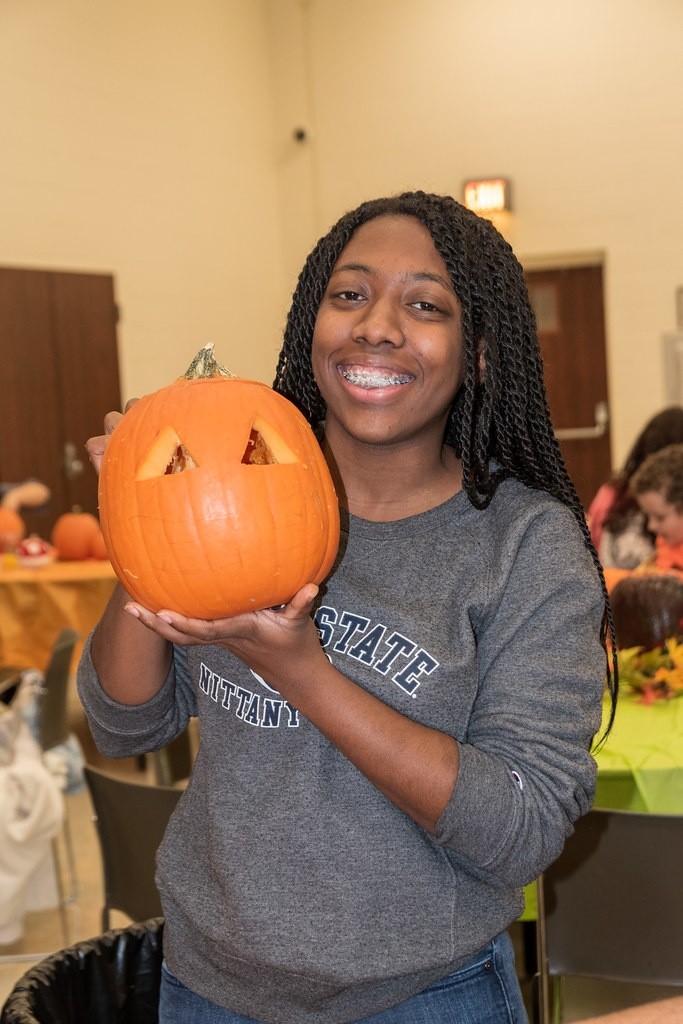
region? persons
[76,191,618,1024]
[588,402,683,578]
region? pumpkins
[53,512,104,562]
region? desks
[0,558,119,671]
[514,684,683,923]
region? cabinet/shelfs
[0,268,122,544]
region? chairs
[82,766,187,934]
[535,810,683,1023]
[0,627,78,958]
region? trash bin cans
[1,916,165,1024]
